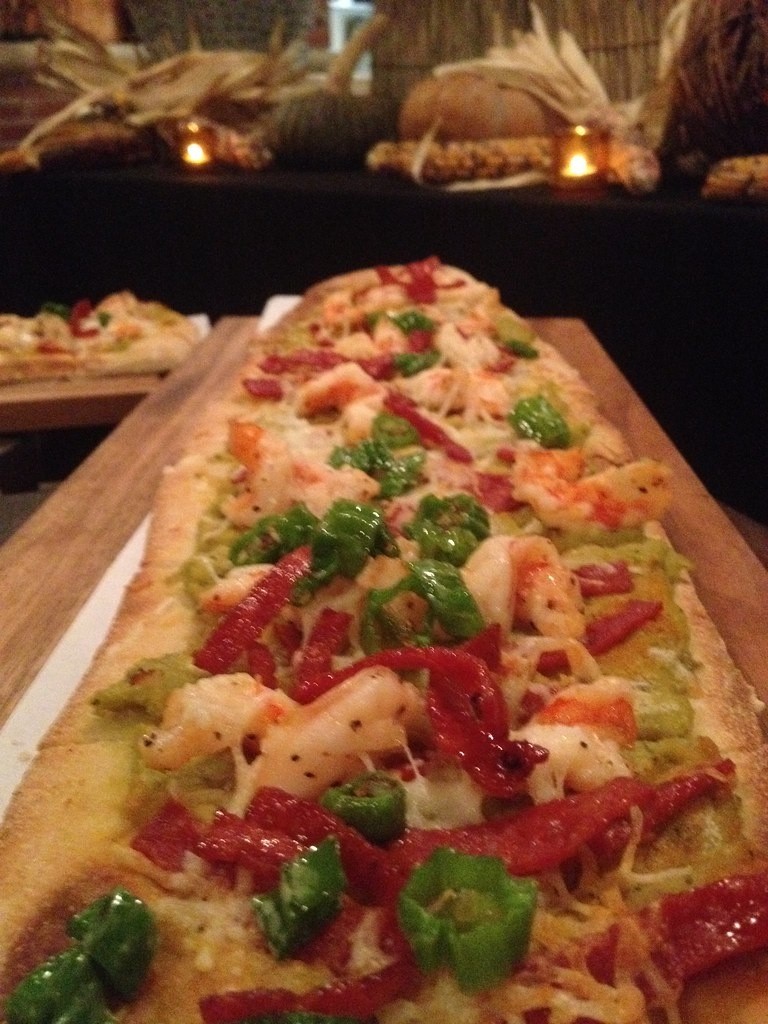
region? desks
[0,162,768,522]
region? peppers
[6,306,566,1024]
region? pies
[0,260,768,1022]
